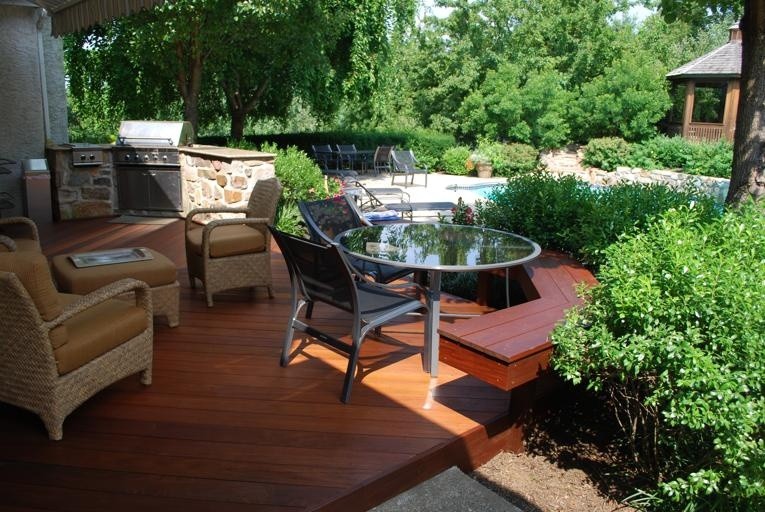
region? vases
[476,162,493,178]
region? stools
[51,246,182,329]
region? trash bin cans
[20,158,54,225]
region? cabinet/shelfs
[117,166,181,210]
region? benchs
[435,249,602,393]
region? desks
[333,223,541,377]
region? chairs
[0,253,153,440]
[311,143,428,189]
